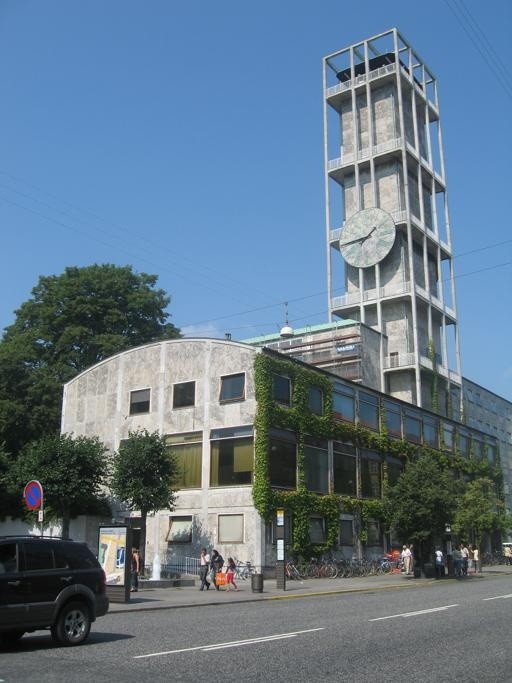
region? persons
[399,542,480,578]
[130,546,141,592]
[225,557,239,593]
[208,549,224,590]
[504,545,512,565]
[199,548,210,591]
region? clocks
[339,206,396,269]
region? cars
[0,535,109,646]
[501,542,512,551]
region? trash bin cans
[251,574,264,593]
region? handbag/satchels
[214,573,226,586]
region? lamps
[279,303,294,338]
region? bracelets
[137,568,139,569]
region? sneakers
[199,583,239,593]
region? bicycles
[285,557,392,579]
[481,551,512,565]
[234,557,257,580]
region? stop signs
[23,480,43,511]
